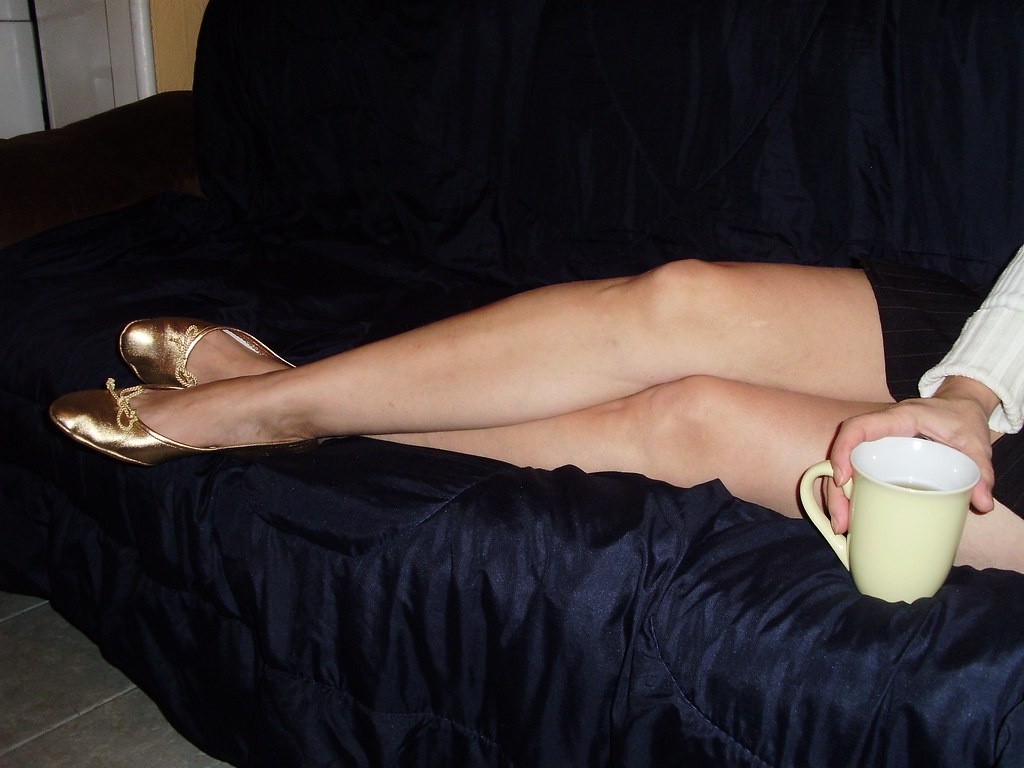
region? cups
[799,437,981,603]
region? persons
[49,242,1024,577]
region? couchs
[0,0,1024,768]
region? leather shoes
[48,383,339,465]
[118,318,299,389]
